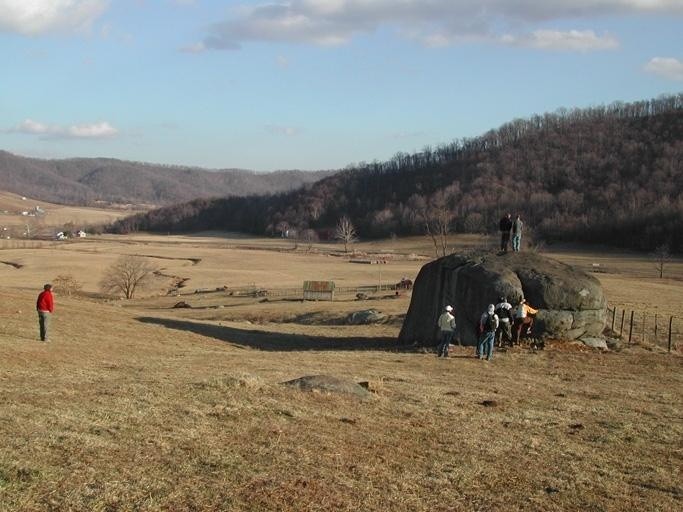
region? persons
[512,214,523,251]
[436,305,457,357]
[476,312,487,355]
[37,284,54,342]
[476,303,499,361]
[500,212,512,251]
[511,298,539,345]
[494,296,515,348]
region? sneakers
[478,356,493,360]
[438,354,450,358]
[41,337,50,341]
[497,330,532,347]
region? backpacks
[484,311,497,333]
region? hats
[444,305,454,311]
